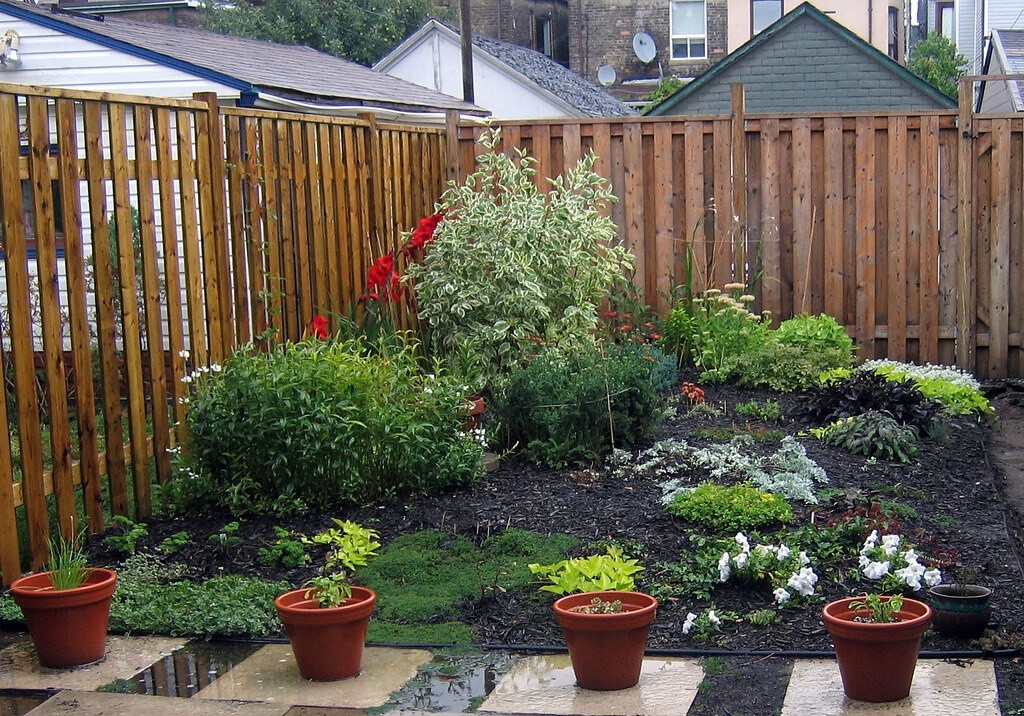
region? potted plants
[826,591,933,703]
[928,548,994,643]
[274,517,381,682]
[10,516,118,667]
[553,587,658,691]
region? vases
[456,394,486,441]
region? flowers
[855,528,943,591]
[305,207,460,351]
[859,356,1001,441]
[675,530,819,640]
